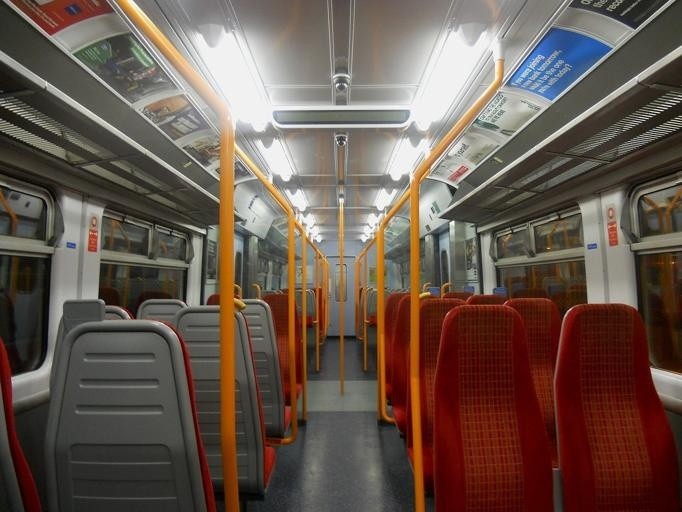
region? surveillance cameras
[334,74,351,91]
[335,134,348,147]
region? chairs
[47,315,217,512]
[51,285,321,501]
[430,301,555,511]
[357,287,564,480]
[1,338,44,510]
[548,301,677,511]
